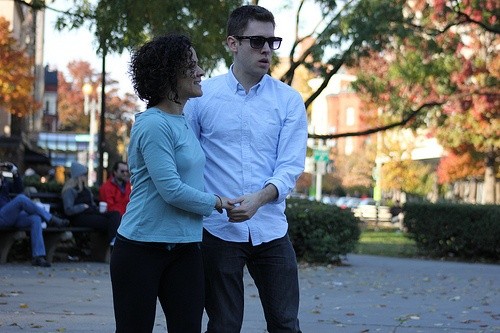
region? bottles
[99,201,107,213]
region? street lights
[83,83,102,186]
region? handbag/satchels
[35,203,51,229]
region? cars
[322,196,375,208]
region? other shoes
[49,216,69,227]
[32,256,50,266]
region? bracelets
[214,194,222,210]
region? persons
[100,161,131,216]
[183,4,308,333]
[110,34,236,333]
[0,162,69,267]
[324,189,374,208]
[62,162,120,261]
[389,200,404,232]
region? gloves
[6,162,17,173]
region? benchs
[360,205,393,232]
[0,193,112,264]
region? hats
[70,162,87,178]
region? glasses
[232,36,282,50]
